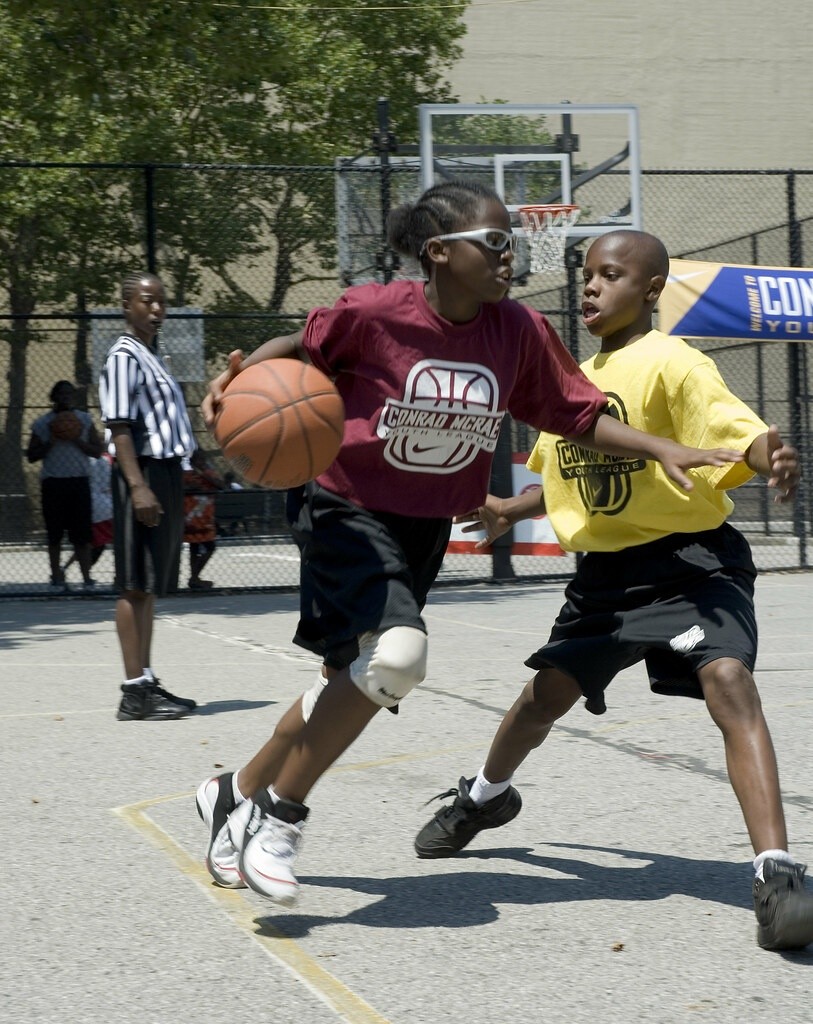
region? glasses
[418,227,518,257]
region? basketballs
[211,361,345,489]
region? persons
[411,230,813,954]
[192,184,747,908]
[26,270,229,723]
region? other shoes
[188,577,215,587]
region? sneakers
[194,772,247,888]
[225,784,309,907]
[752,857,813,950]
[116,676,197,721]
[414,776,522,857]
